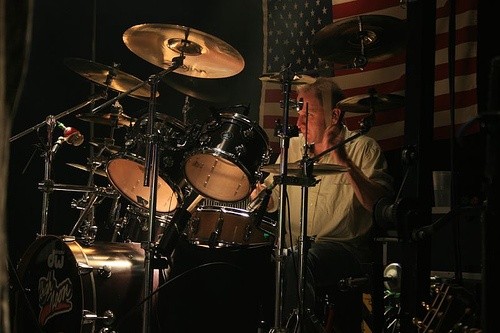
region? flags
[259,0,478,155]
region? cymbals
[123,24,245,79]
[311,14,411,63]
[259,161,351,177]
[64,56,159,102]
[335,92,411,113]
[73,112,140,129]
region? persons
[247,75,394,333]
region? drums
[106,113,187,213]
[184,110,271,206]
[189,203,281,255]
[115,203,181,259]
[9,234,174,333]
